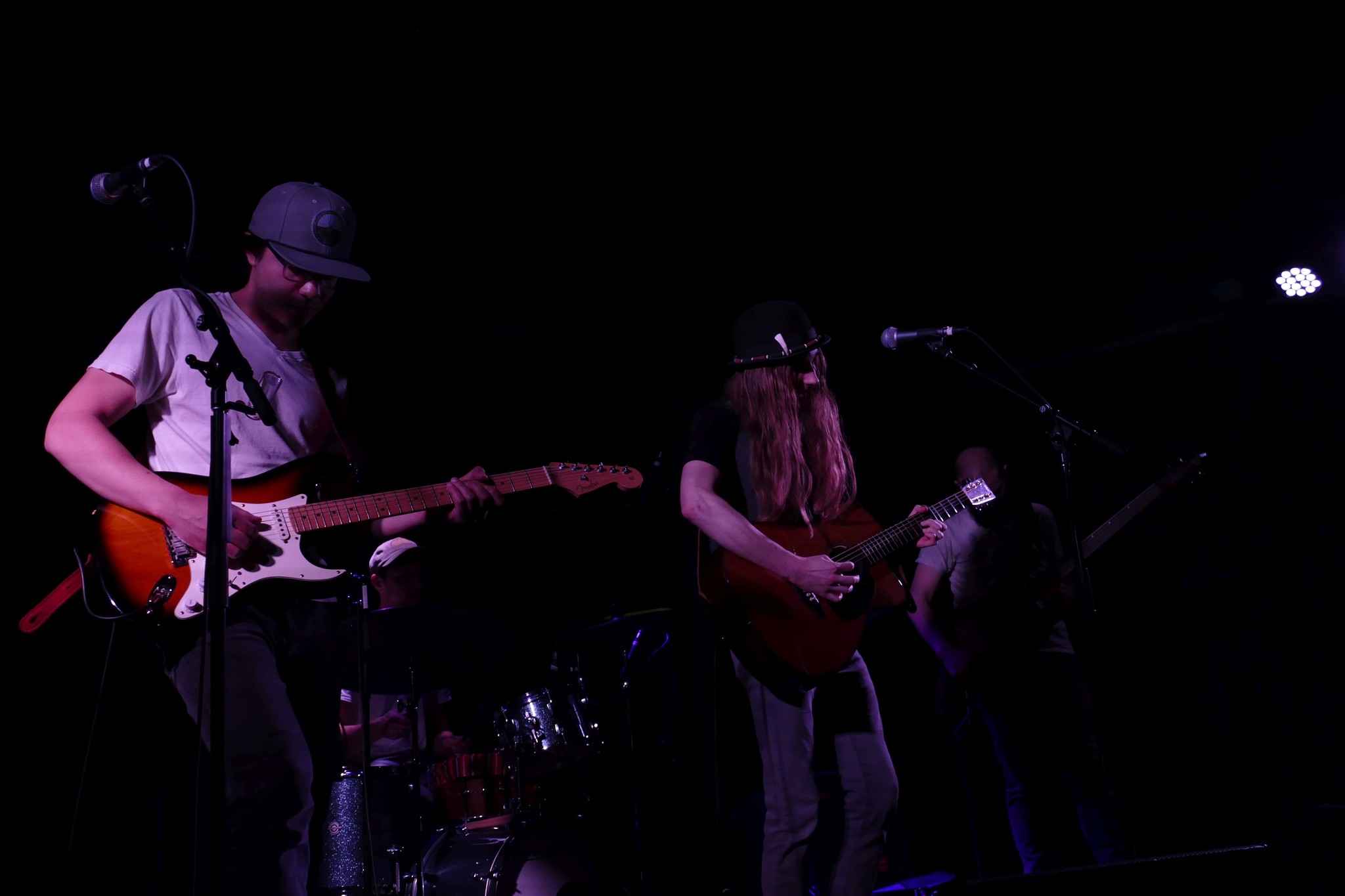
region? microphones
[880,326,967,350]
[628,629,644,661]
[90,155,167,205]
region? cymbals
[571,607,674,645]
[319,604,512,697]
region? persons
[44,182,503,895]
[680,314,948,896]
[906,445,1112,886]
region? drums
[317,765,395,894]
[403,819,586,896]
[440,747,543,831]
[491,687,590,767]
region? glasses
[267,245,339,290]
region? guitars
[700,475,997,710]
[82,455,648,641]
[937,447,1214,687]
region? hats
[249,182,372,283]
[730,301,831,365]
